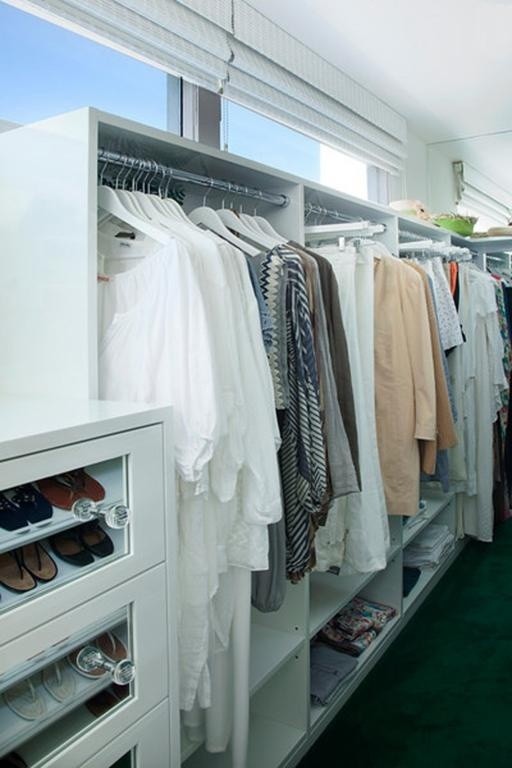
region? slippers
[41,659,77,702]
[11,486,53,525]
[0,492,28,531]
[77,524,114,558]
[94,630,128,664]
[66,646,110,680]
[60,468,106,502]
[111,682,132,701]
[85,691,118,718]
[48,530,96,566]
[18,542,58,582]
[36,477,82,512]
[3,677,48,721]
[0,751,28,768]
[0,552,37,593]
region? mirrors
[485,251,512,523]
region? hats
[390,199,430,220]
[487,221,512,235]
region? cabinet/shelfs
[303,147,402,763]
[0,404,182,767]
[94,118,309,767]
[450,216,483,565]
[397,186,450,642]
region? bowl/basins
[435,218,475,237]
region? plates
[488,228,512,234]
